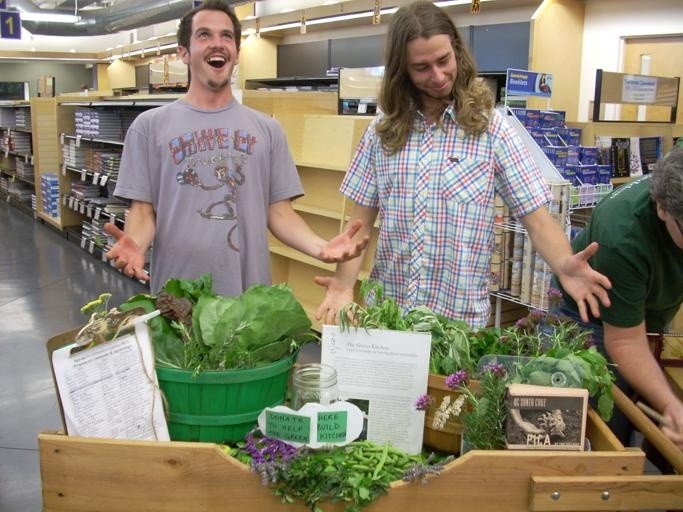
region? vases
[460,432,473,457]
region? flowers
[416,362,510,450]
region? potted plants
[339,279,513,454]
[120,274,321,444]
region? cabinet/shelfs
[492,182,614,312]
[0,97,56,219]
[37,403,645,512]
[58,98,178,287]
[267,160,380,332]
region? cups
[290,362,339,414]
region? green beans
[341,440,419,481]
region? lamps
[157,39,160,55]
[471,0,480,16]
[299,10,306,34]
[373,0,381,24]
[141,44,144,58]
[21,12,81,23]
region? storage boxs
[503,382,590,452]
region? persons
[309,1,614,339]
[543,141,683,474]
[99,1,373,310]
